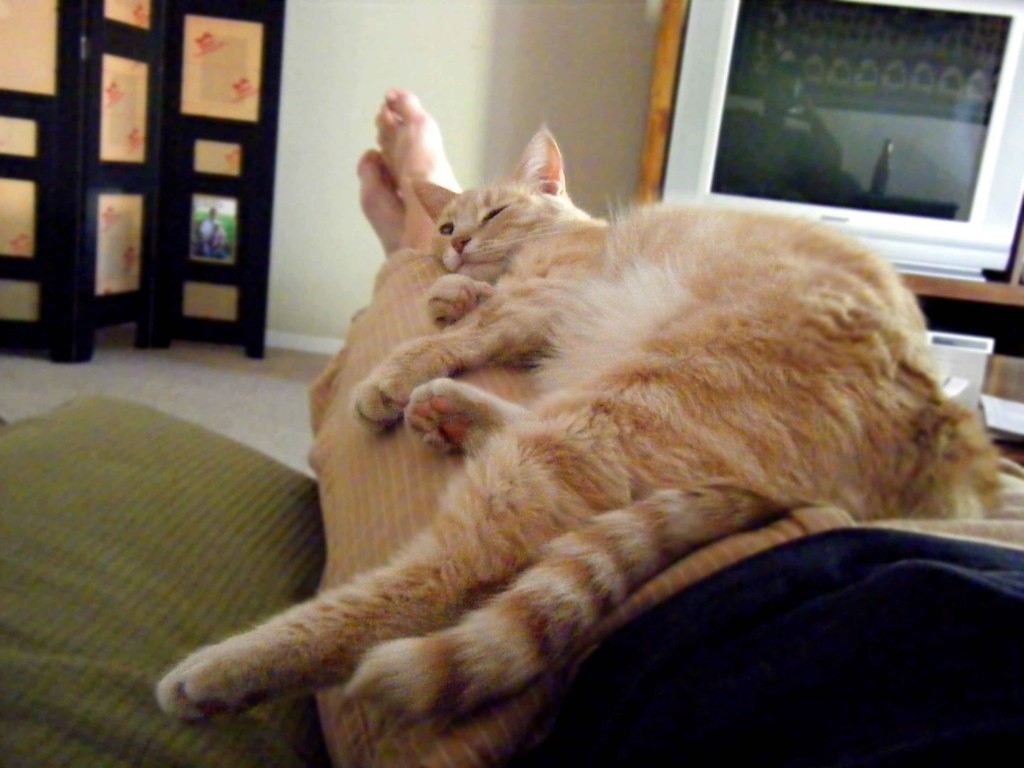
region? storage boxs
[927,328,995,408]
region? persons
[196,206,230,260]
[308,87,1024,768]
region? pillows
[0,394,328,768]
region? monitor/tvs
[657,0,1024,286]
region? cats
[156,125,1007,719]
